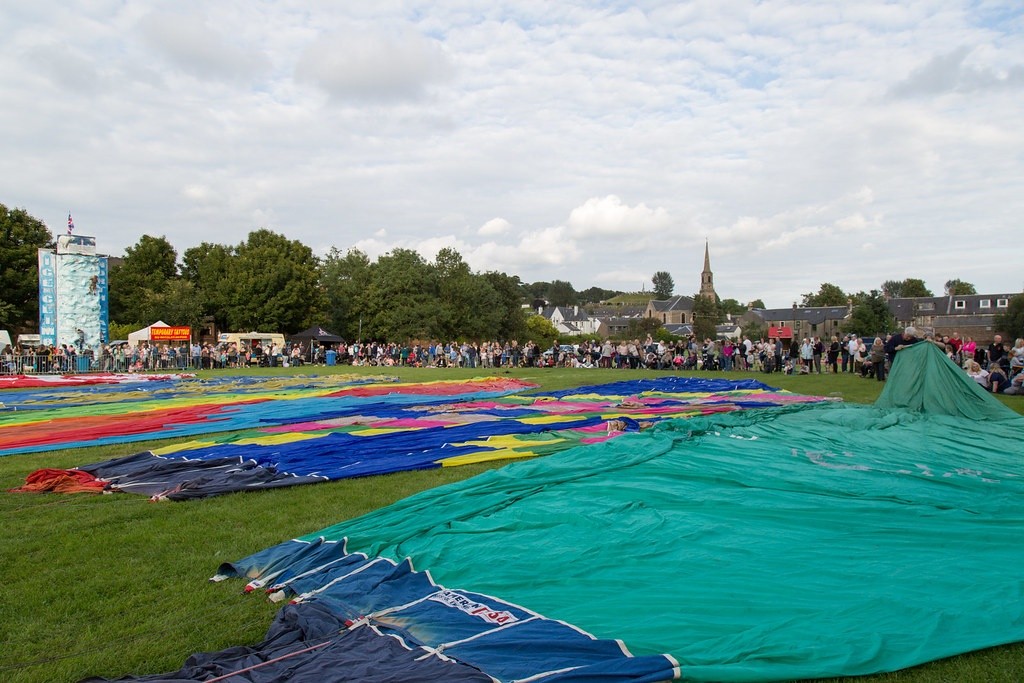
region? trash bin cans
[76,355,89,374]
[325,350,337,366]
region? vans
[0,330,14,371]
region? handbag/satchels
[859,350,869,358]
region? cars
[110,340,128,350]
[862,336,875,349]
[542,345,575,362]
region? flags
[68,214,74,234]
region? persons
[0,327,1024,395]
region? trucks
[214,330,286,363]
[16,334,40,355]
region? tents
[290,325,345,343]
[124,321,191,347]
